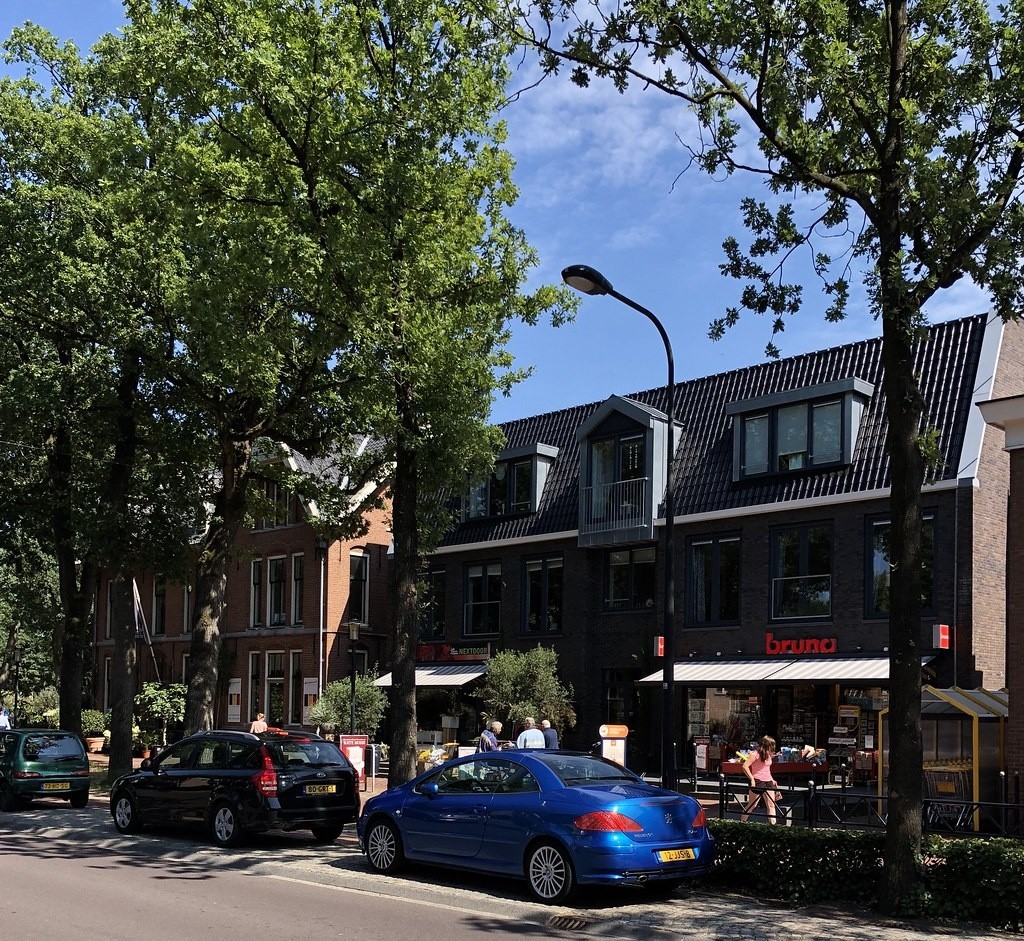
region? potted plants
[480,709,497,729]
[440,708,465,728]
[81,709,106,752]
[133,682,188,758]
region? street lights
[342,613,370,735]
[561,265,676,790]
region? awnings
[369,666,489,685]
[634,656,938,683]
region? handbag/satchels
[775,783,782,800]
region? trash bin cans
[364,745,381,775]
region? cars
[0,729,91,810]
[356,747,716,905]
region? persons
[250,713,267,733]
[474,720,502,779]
[741,735,778,824]
[0,708,11,729]
[506,717,545,749]
[541,720,559,749]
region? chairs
[419,742,459,782]
[214,746,226,763]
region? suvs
[110,730,361,847]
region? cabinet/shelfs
[828,705,862,786]
[782,731,805,745]
